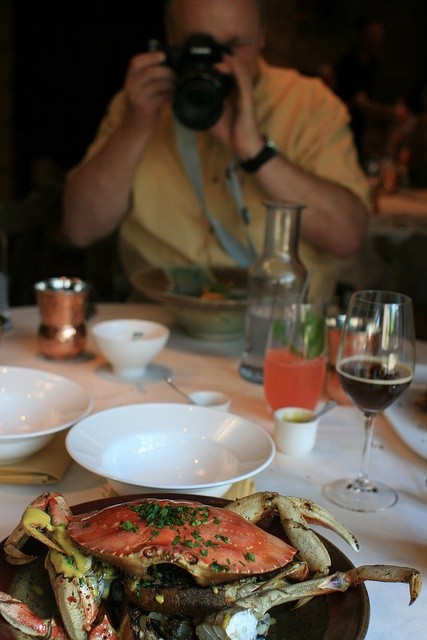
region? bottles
[238,201,326,384]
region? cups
[190,391,233,412]
[263,300,329,415]
[275,406,319,456]
[26,276,96,360]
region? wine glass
[321,289,417,515]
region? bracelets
[241,134,281,172]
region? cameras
[132,32,251,133]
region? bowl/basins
[0,365,94,467]
[65,402,276,499]
[91,319,171,380]
[160,286,249,342]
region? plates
[383,362,427,461]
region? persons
[61,0,372,315]
